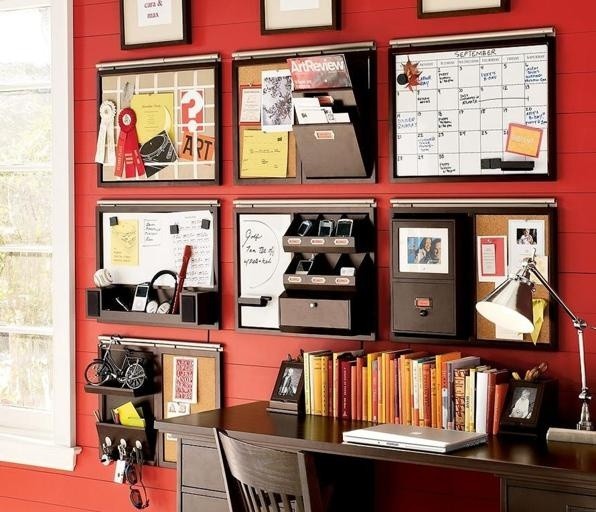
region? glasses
[126,448,150,511]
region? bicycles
[84,333,148,389]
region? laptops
[342,423,488,454]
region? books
[299,346,515,437]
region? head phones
[146,270,179,314]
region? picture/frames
[269,360,303,411]
[393,222,453,279]
[500,378,544,427]
[121,1,191,49]
[261,0,341,35]
[417,0,511,19]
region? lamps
[476,257,596,445]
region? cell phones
[336,219,354,237]
[296,220,313,237]
[295,260,314,275]
[318,220,335,236]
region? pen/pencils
[287,353,292,362]
[512,362,549,381]
[298,348,303,360]
[111,409,121,425]
[93,409,102,422]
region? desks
[154,401,596,512]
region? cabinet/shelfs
[279,213,369,335]
[496,476,596,512]
[391,280,457,336]
[175,437,301,512]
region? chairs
[213,427,335,512]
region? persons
[419,239,442,264]
[508,389,534,420]
[518,229,535,244]
[414,237,432,264]
